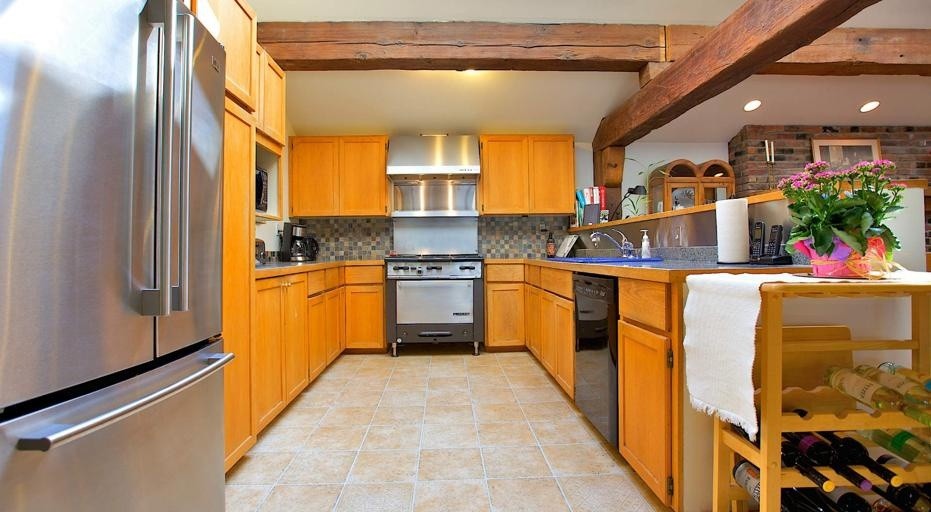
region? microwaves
[256,166,269,213]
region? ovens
[386,278,483,357]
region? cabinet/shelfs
[647,158,735,214]
[223,97,257,479]
[476,134,575,214]
[257,261,387,439]
[255,44,289,149]
[285,137,386,216]
[191,0,258,113]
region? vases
[794,235,889,279]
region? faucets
[590,227,635,258]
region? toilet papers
[714,197,751,265]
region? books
[575,184,608,223]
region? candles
[764,140,769,162]
[769,141,774,162]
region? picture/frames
[810,136,883,170]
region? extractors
[386,136,482,218]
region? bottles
[546,232,556,258]
[730,361,931,511]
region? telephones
[758,225,792,264]
[748,221,765,263]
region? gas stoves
[384,253,483,260]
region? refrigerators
[0,0,234,512]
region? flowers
[779,160,908,262]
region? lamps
[608,184,650,221]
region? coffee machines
[281,222,309,262]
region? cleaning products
[640,229,651,259]
